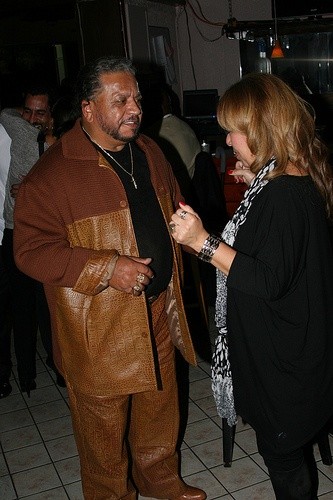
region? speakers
[183,89,218,119]
[271,0,333,20]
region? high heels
[21,381,36,397]
[0,383,12,398]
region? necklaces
[82,126,137,190]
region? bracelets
[197,232,221,263]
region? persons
[169,70,333,500]
[135,67,203,201]
[0,76,66,399]
[14,60,206,500]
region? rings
[134,285,141,292]
[137,272,144,283]
[169,224,176,234]
[180,211,188,219]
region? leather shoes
[169,486,207,500]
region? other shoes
[46,358,67,388]
